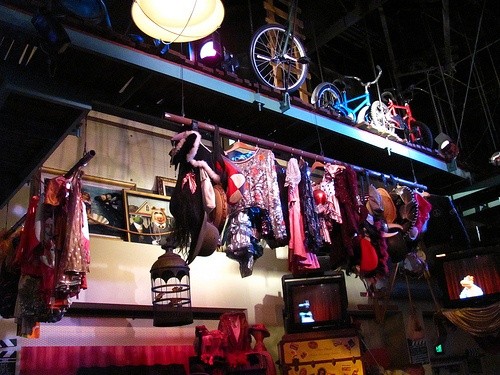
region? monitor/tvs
[281,270,351,334]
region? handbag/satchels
[404,273,438,341]
[357,261,400,326]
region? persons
[298,300,315,323]
[82,190,122,231]
[459,275,483,299]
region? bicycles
[309,65,383,128]
[370,84,434,149]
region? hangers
[420,189,429,197]
[224,139,258,157]
[392,183,403,192]
[310,160,325,175]
[299,156,306,168]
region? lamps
[32,10,71,54]
[434,131,459,159]
[131,0,225,44]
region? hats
[366,187,396,223]
[186,211,220,265]
[214,185,227,228]
[386,185,418,239]
[408,191,431,240]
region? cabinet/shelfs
[277,322,368,375]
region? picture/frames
[122,190,191,245]
[29,166,136,240]
[156,176,179,195]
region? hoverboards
[248,0,311,92]
